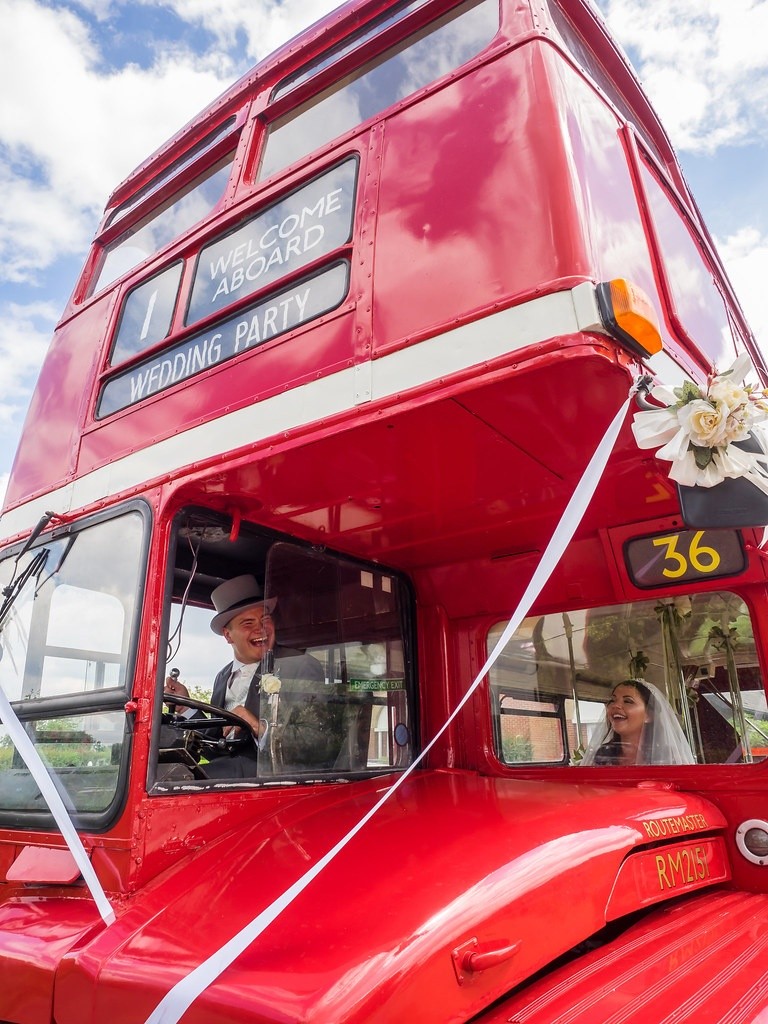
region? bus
[1,0,767,1024]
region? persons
[162,575,341,780]
[579,678,696,766]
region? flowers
[654,594,694,626]
[568,745,587,767]
[255,672,282,694]
[630,650,650,672]
[708,625,742,652]
[634,351,768,489]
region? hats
[210,574,278,635]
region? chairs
[326,682,375,771]
